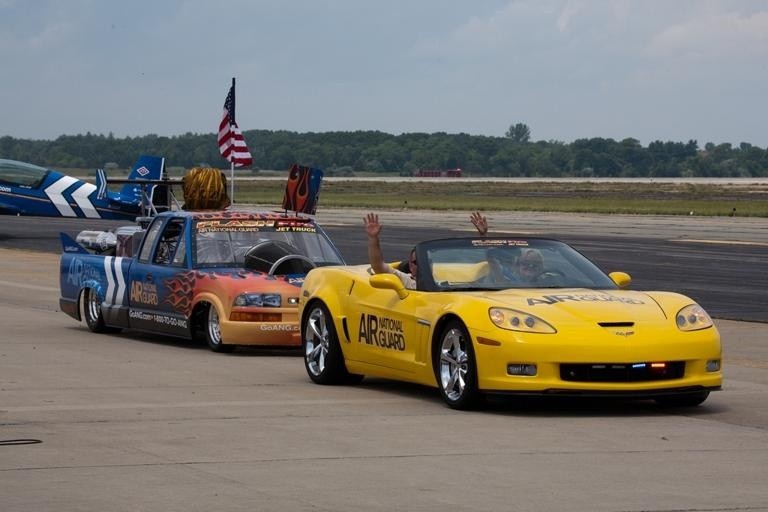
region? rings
[478,220,480,224]
[476,222,478,225]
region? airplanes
[0,155,170,219]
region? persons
[470,211,544,286]
[362,212,440,291]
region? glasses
[522,259,542,269]
[412,259,432,266]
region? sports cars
[298,239,724,410]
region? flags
[217,79,255,168]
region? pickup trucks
[60,206,347,352]
